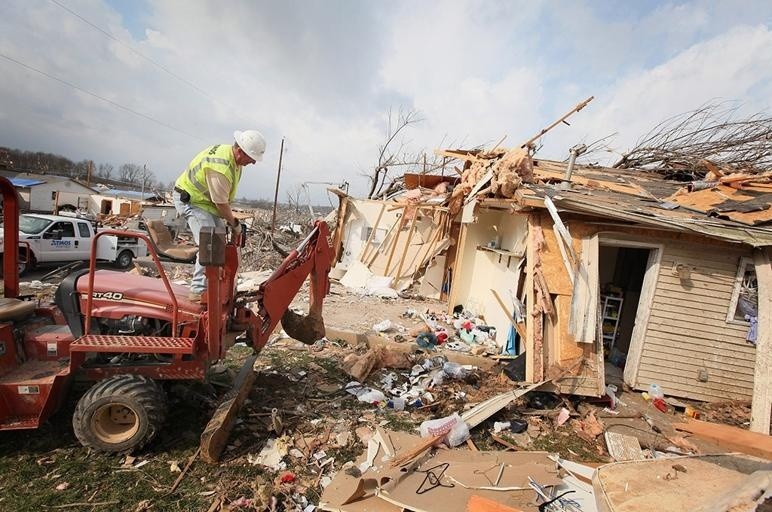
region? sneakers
[189,292,201,300]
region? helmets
[235,130,266,161]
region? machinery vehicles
[0,174,337,462]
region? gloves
[227,218,243,235]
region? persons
[172,128,267,302]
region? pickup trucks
[0,213,148,277]
